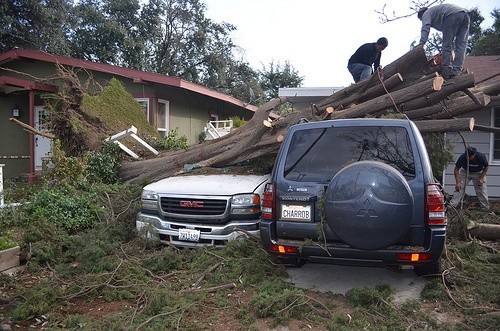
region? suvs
[259,113,447,279]
[135,173,272,251]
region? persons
[446,147,490,210]
[347,37,388,84]
[417,4,470,79]
